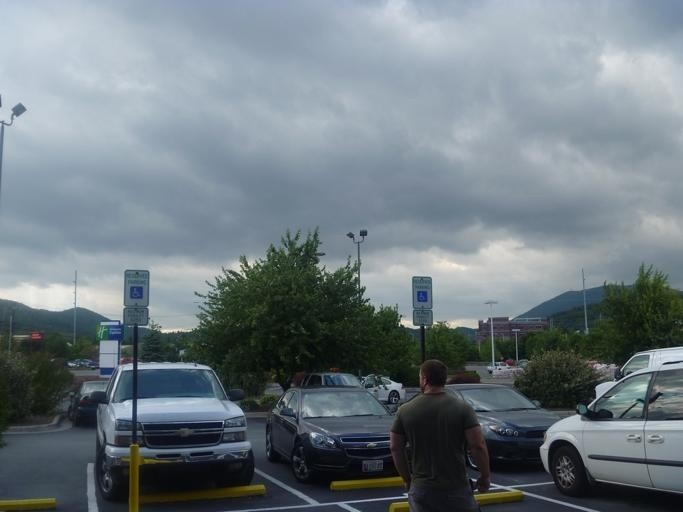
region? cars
[538,368,683,497]
[266,387,409,482]
[66,380,107,427]
[299,372,362,387]
[407,383,561,471]
[358,374,406,404]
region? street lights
[345,228,370,305]
[484,299,499,366]
[0,89,29,211]
[510,328,522,361]
[580,265,587,330]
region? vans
[592,346,683,401]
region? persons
[388,357,493,511]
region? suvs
[95,361,254,499]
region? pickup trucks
[486,361,512,374]
